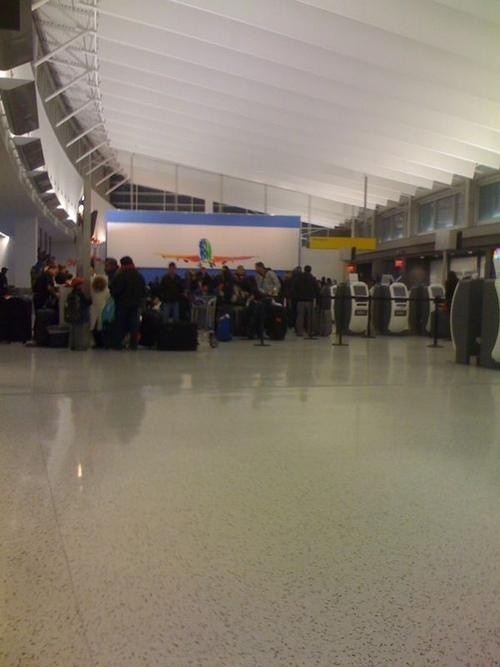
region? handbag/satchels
[47,332,69,350]
[155,320,198,352]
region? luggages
[231,304,250,337]
[1,294,32,344]
[313,306,333,338]
[216,318,232,342]
[270,304,289,341]
[138,308,160,348]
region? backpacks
[64,295,82,323]
[100,297,116,328]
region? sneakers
[70,343,139,352]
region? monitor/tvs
[77,210,97,239]
[393,286,406,296]
[354,286,367,296]
[333,287,337,294]
[432,286,444,297]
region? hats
[70,276,87,288]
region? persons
[1,252,336,351]
[444,270,460,311]
[374,270,402,286]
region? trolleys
[190,293,221,349]
